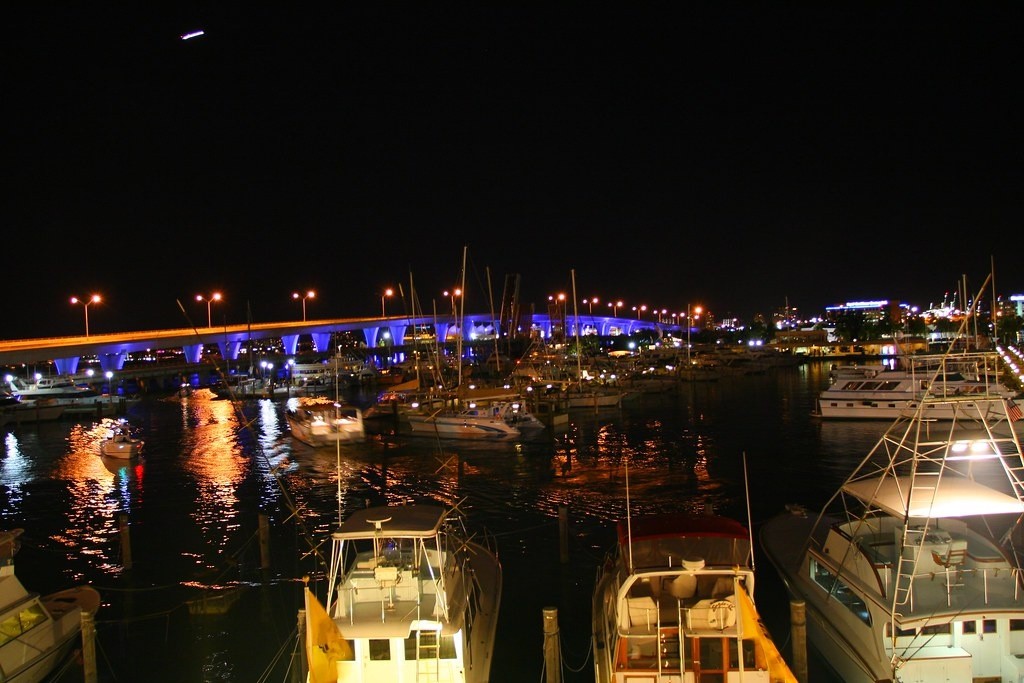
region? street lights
[381,289,393,316]
[69,293,102,337]
[607,301,623,317]
[293,291,315,322]
[548,293,565,314]
[632,305,647,320]
[652,309,667,324]
[444,290,462,314]
[582,297,598,315]
[671,312,685,326]
[196,292,220,327]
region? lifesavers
[709,600,736,629]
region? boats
[0,528,101,683]
[587,453,799,683]
[761,272,1024,683]
[300,356,504,683]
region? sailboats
[2,245,1024,444]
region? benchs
[685,599,736,629]
[712,578,747,598]
[627,589,657,625]
[852,532,1005,585]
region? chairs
[670,575,697,607]
[373,567,400,612]
